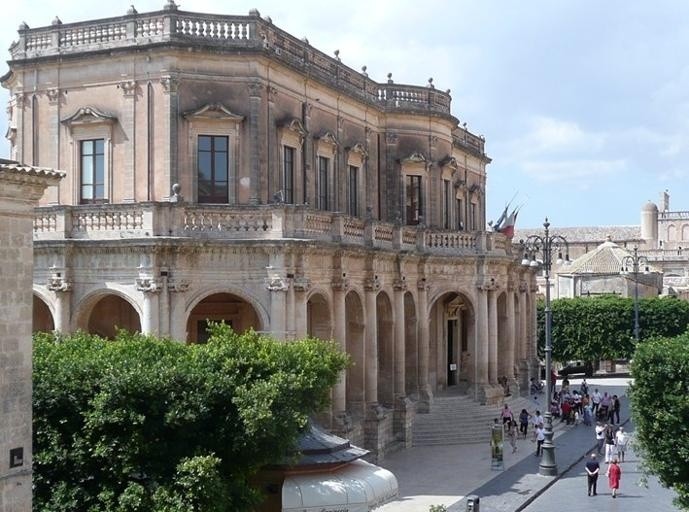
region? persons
[492,367,629,464]
[607,458,621,497]
[585,453,600,496]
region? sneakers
[593,493,597,496]
[612,494,616,499]
[588,493,590,496]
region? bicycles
[528,377,545,393]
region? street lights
[518,215,572,477]
[617,243,651,342]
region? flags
[500,213,515,240]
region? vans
[556,360,594,377]
